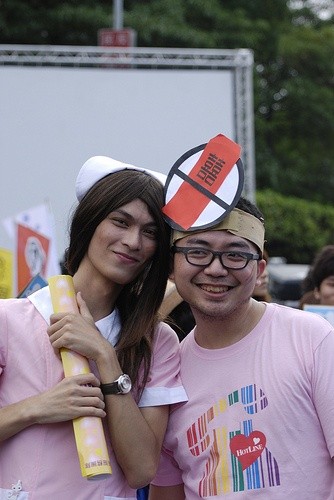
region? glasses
[173,246,259,270]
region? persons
[1,154,191,500]
[296,246,334,311]
[146,192,334,500]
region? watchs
[98,373,133,396]
